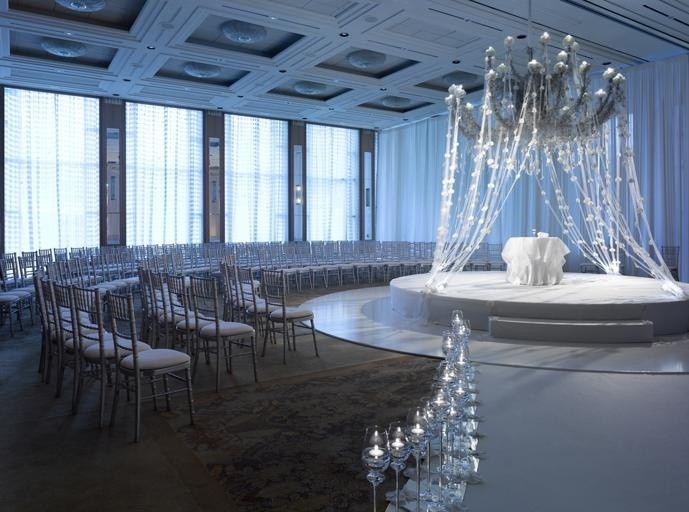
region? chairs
[0,239,506,443]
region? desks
[509,236,562,285]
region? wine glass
[361,309,480,512]
